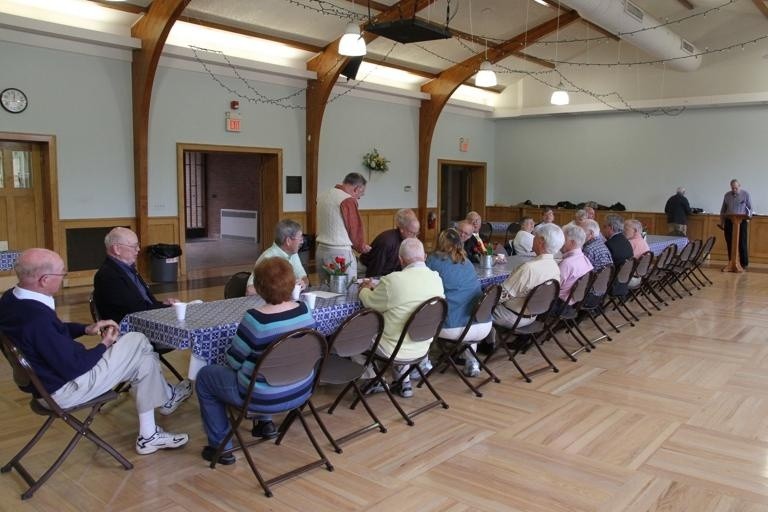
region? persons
[720,178,755,269]
[244,217,310,299]
[663,186,705,237]
[347,235,446,399]
[194,257,317,468]
[425,207,650,377]
[90,225,189,355]
[0,247,193,454]
[315,169,374,288]
[358,203,423,279]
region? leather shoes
[478,343,496,352]
[251,422,277,440]
[201,446,235,465]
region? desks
[115,264,506,400]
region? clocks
[0,88,28,113]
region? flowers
[326,254,350,268]
[362,148,391,172]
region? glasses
[112,242,140,248]
[292,237,303,244]
[38,271,67,282]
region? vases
[331,272,351,298]
[369,169,376,185]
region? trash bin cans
[147,243,182,282]
[297,234,311,264]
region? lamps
[474,38,497,88]
[338,0,367,57]
[551,67,569,105]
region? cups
[304,293,317,310]
[497,253,504,258]
[642,231,646,240]
[172,301,188,321]
[292,284,301,302]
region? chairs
[208,327,335,495]
[281,308,387,447]
[350,296,451,425]
[412,283,504,397]
[85,294,190,399]
[1,336,133,497]
[223,272,258,300]
[477,221,716,381]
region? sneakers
[352,377,389,396]
[159,378,192,416]
[135,426,188,456]
[409,360,432,381]
[390,381,412,397]
[462,361,480,377]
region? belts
[502,303,536,318]
